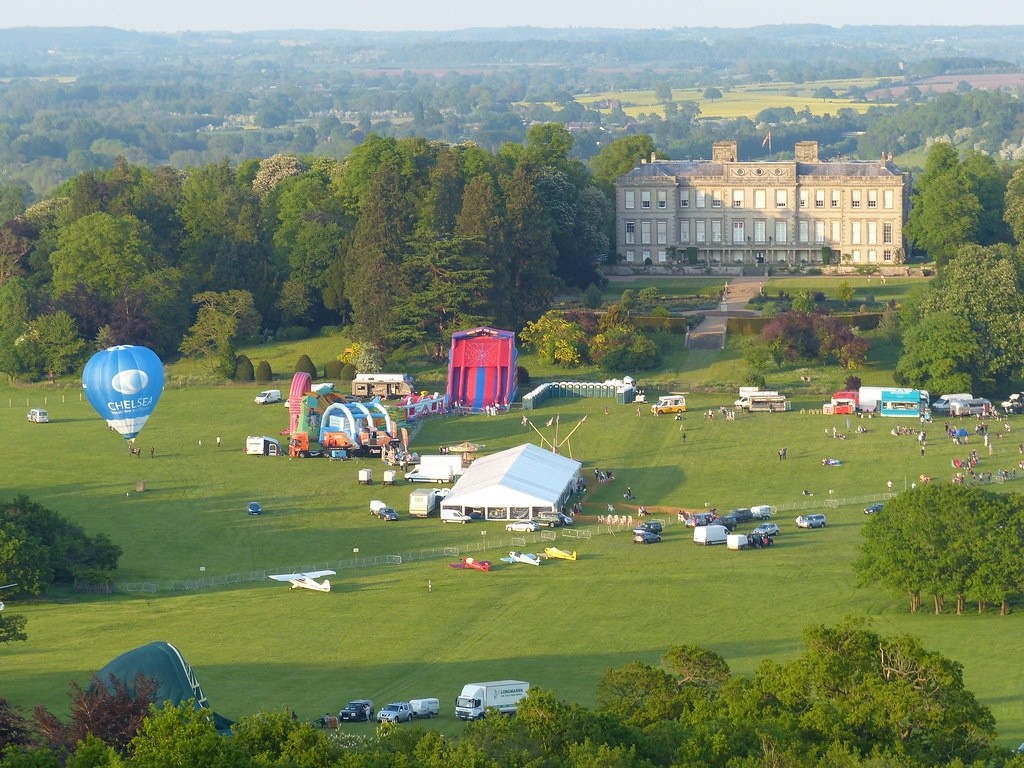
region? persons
[778,445,787,461]
[825,426,845,440]
[759,281,763,295]
[802,489,814,496]
[363,704,370,725]
[682,433,686,442]
[128,445,141,458]
[748,531,769,550]
[769,402,772,413]
[291,710,297,721]
[400,460,408,472]
[821,457,840,466]
[325,713,330,730]
[561,467,648,517]
[636,407,641,417]
[857,425,866,433]
[708,405,729,420]
[216,436,221,447]
[880,274,885,284]
[890,404,1024,487]
[906,267,910,277]
[151,446,155,458]
[725,281,729,296]
[439,445,449,454]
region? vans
[441,509,471,524]
[404,465,453,484]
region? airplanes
[448,546,576,573]
[269,570,336,594]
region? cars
[247,502,262,515]
[378,507,400,521]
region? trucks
[734,391,778,409]
[409,488,435,518]
[26,409,49,424]
[455,680,530,722]
[288,432,332,458]
[651,395,686,415]
[246,436,282,456]
[932,394,992,416]
[1001,391,1024,413]
[255,390,281,405]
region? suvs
[633,521,662,544]
[798,514,826,528]
[505,512,573,532]
[339,700,374,723]
[685,505,779,546]
[377,702,413,724]
[863,504,883,515]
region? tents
[78,642,241,733]
[440,442,583,522]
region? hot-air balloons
[82,345,164,453]
[85,642,337,737]
